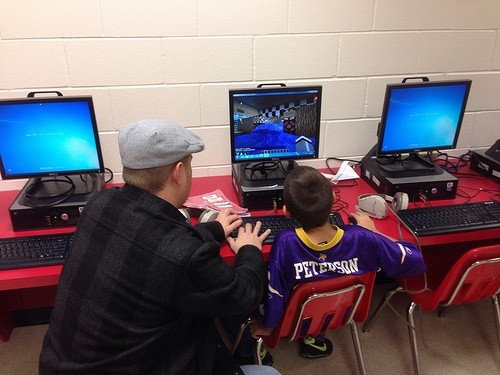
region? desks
[0,159,500,343]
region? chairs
[230,268,377,375]
[363,244,500,375]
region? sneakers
[300,335,333,358]
[255,343,273,366]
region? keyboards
[229,212,344,245]
[396,199,500,236]
[0,233,75,270]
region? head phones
[358,192,409,220]
[177,208,220,225]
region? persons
[233,166,426,366]
[38,119,272,375]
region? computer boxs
[360,155,459,202]
[9,173,105,233]
[470,150,500,184]
[231,164,285,210]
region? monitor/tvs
[377,78,472,172]
[228,86,323,188]
[0,96,104,208]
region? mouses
[347,216,357,225]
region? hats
[117,121,204,168]
